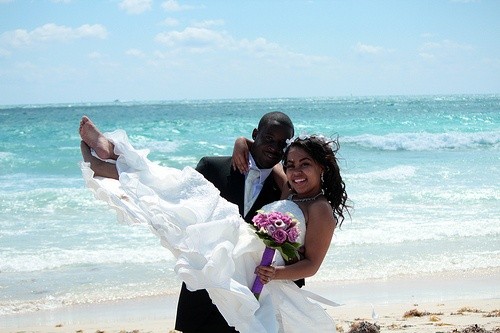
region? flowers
[248,210,301,301]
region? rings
[267,277,271,282]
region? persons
[175,111,305,333]
[79,116,352,333]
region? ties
[244,169,260,218]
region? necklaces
[287,190,324,202]
